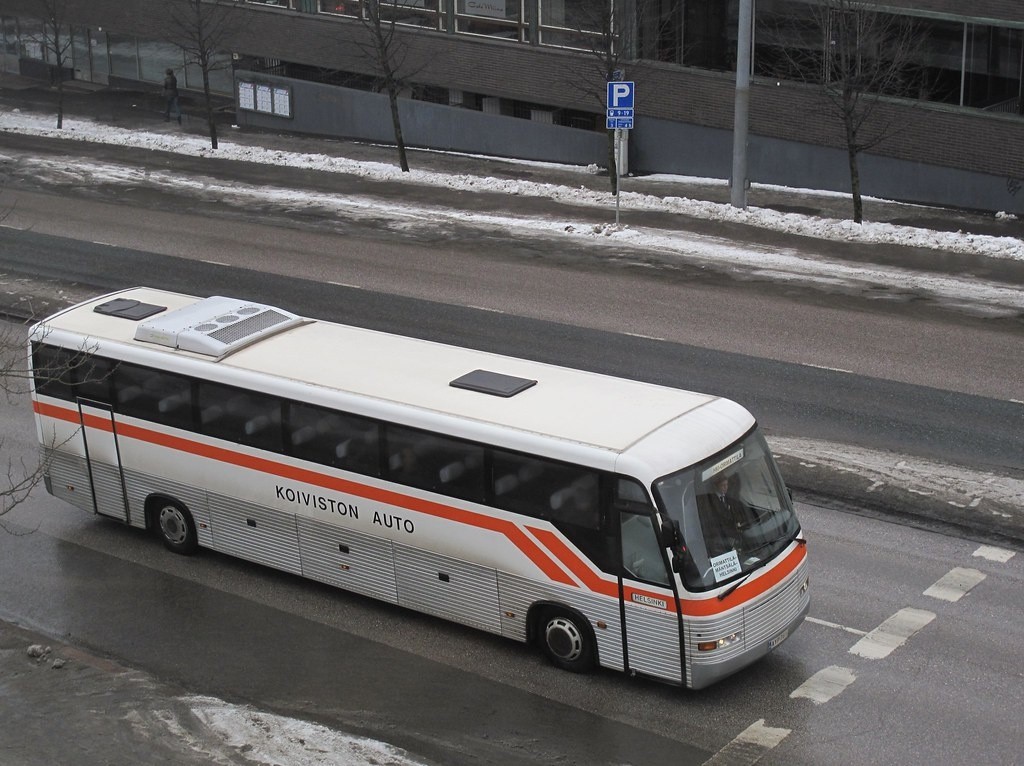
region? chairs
[550,487,575,522]
[271,405,304,432]
[292,425,322,460]
[390,437,441,482]
[202,404,231,436]
[158,394,190,426]
[117,377,165,417]
[182,386,209,412]
[465,454,494,483]
[226,393,256,426]
[317,414,347,450]
[570,475,599,512]
[245,415,281,449]
[335,429,392,473]
[496,474,522,507]
[440,461,473,497]
[622,516,665,582]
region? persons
[160,68,183,125]
[708,474,752,539]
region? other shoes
[165,119,170,122]
[178,116,181,124]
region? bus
[29,286,811,693]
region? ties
[720,496,724,503]
